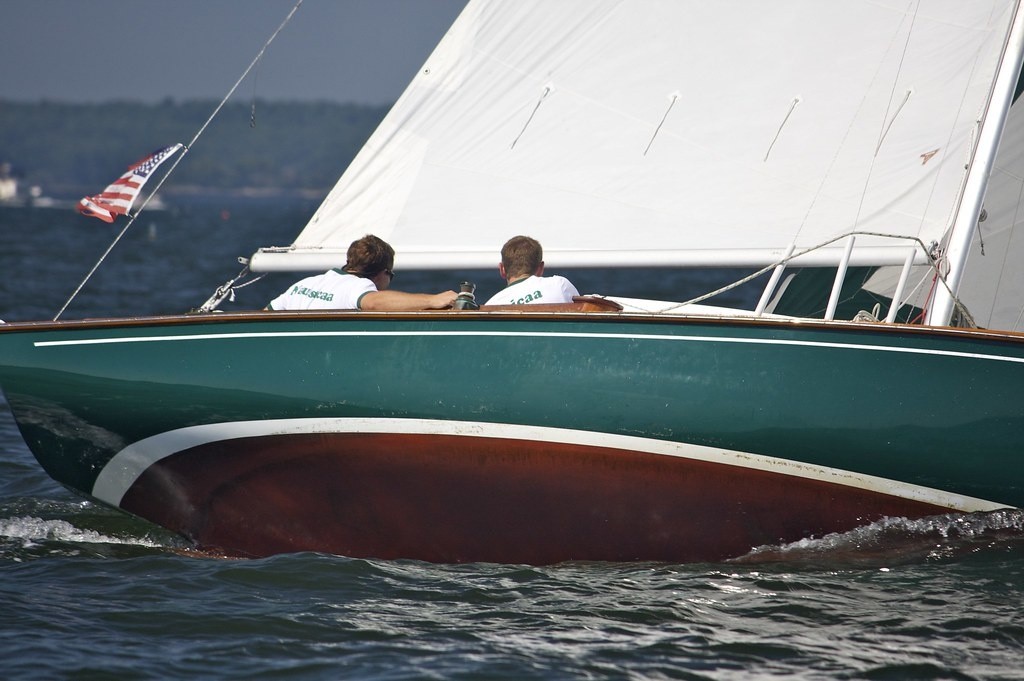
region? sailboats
[0,2,1022,572]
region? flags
[76,142,184,223]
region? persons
[483,234,581,304]
[263,234,460,310]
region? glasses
[387,268,394,281]
